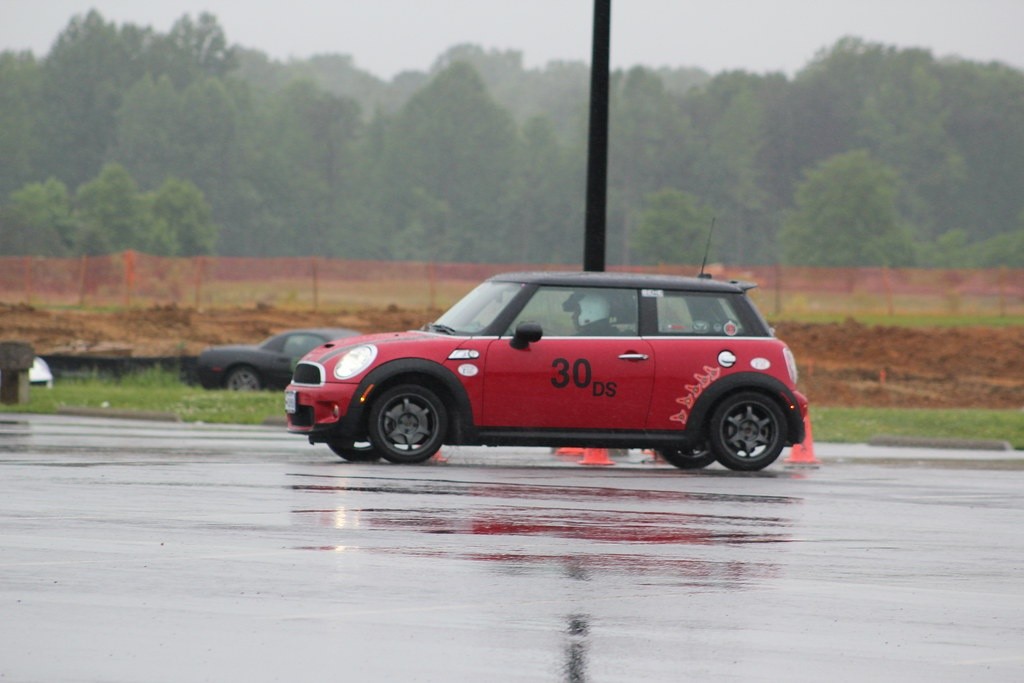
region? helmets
[562,293,612,328]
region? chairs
[608,292,638,337]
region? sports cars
[281,271,810,472]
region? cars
[197,326,365,393]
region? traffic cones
[417,444,449,462]
[555,446,583,455]
[782,411,820,465]
[577,446,616,466]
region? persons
[570,291,621,336]
